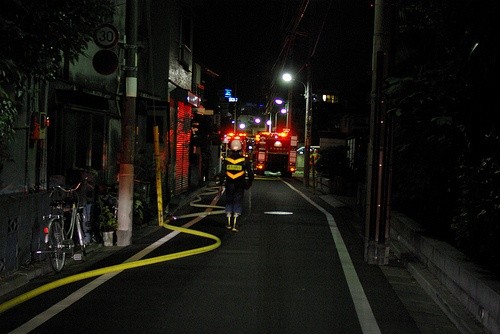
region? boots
[226,217,232,229]
[232,217,240,232]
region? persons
[310,149,320,165]
[217,140,254,231]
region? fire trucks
[253,130,298,178]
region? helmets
[231,140,242,152]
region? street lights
[282,73,310,187]
[275,98,291,129]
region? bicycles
[44,175,94,274]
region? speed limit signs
[93,23,120,49]
